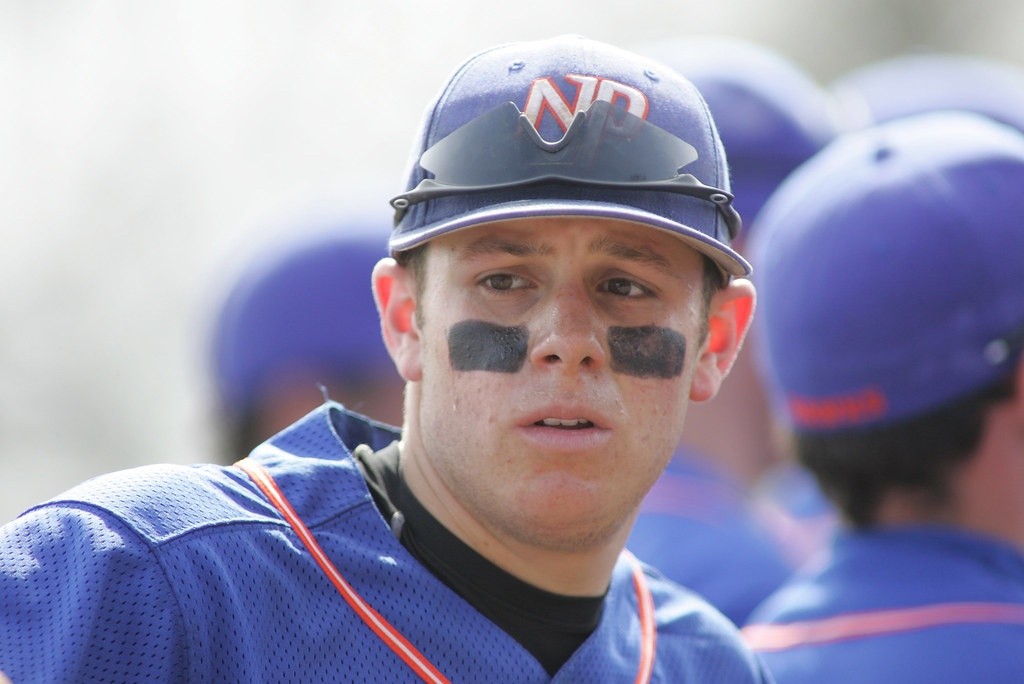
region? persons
[0,35,772,682]
[739,113,1024,683]
[206,43,1023,626]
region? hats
[209,226,397,415]
[827,55,1024,134]
[389,33,753,278]
[645,46,840,231]
[740,110,1024,432]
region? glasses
[389,99,742,242]
